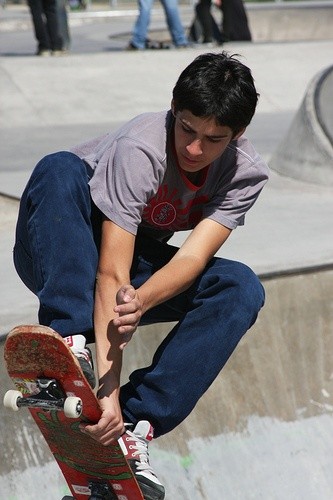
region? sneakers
[51,50,60,56]
[62,335,96,389]
[38,47,50,57]
[117,420,165,500]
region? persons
[129,0,187,51]
[213,1,251,41]
[14,50,270,499]
[24,0,69,56]
[189,0,228,42]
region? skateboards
[4,324,144,500]
